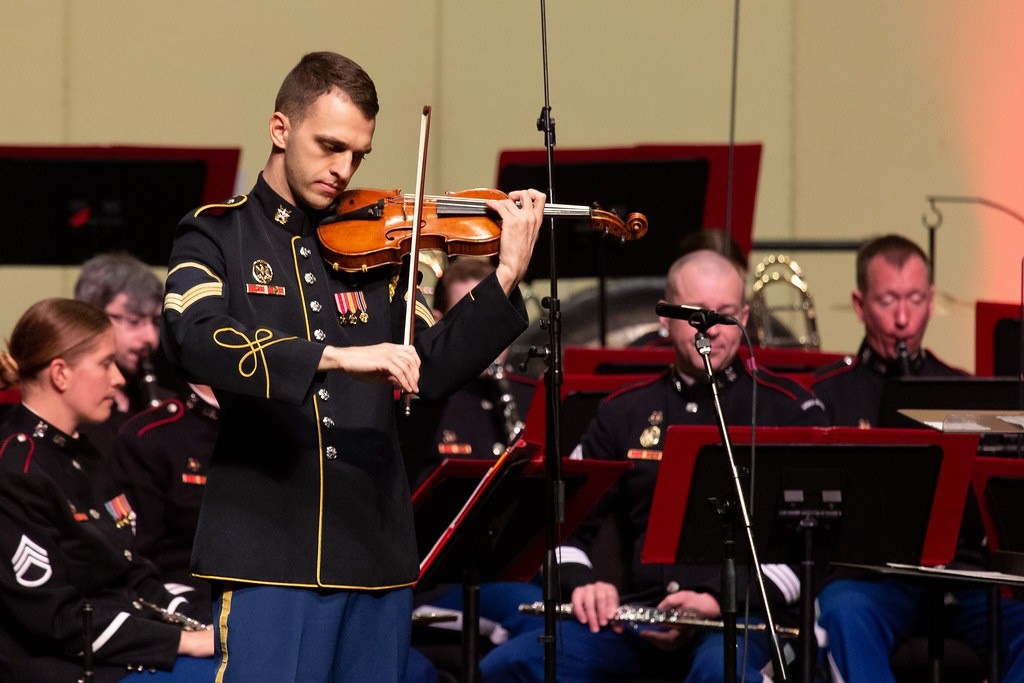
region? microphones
[655,303,738,325]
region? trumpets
[751,253,822,354]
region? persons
[482,251,852,683]
[0,257,221,683]
[158,52,547,683]
[810,234,1023,683]
[407,259,571,682]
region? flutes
[139,597,210,632]
[515,597,802,640]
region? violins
[313,186,651,279]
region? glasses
[103,310,162,329]
